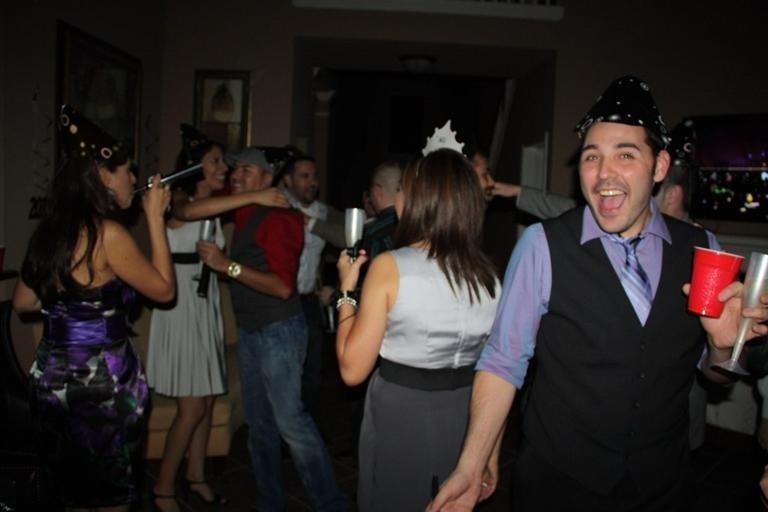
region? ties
[614,232,653,326]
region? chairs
[130,272,251,461]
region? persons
[6,125,728,508]
[423,68,768,510]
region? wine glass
[343,206,365,266]
[708,248,767,377]
[190,217,215,281]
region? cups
[687,243,745,318]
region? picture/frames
[188,64,253,165]
[48,12,145,187]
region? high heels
[148,489,185,512]
[184,478,228,507]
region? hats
[575,77,664,140]
[58,104,130,172]
[224,149,272,170]
